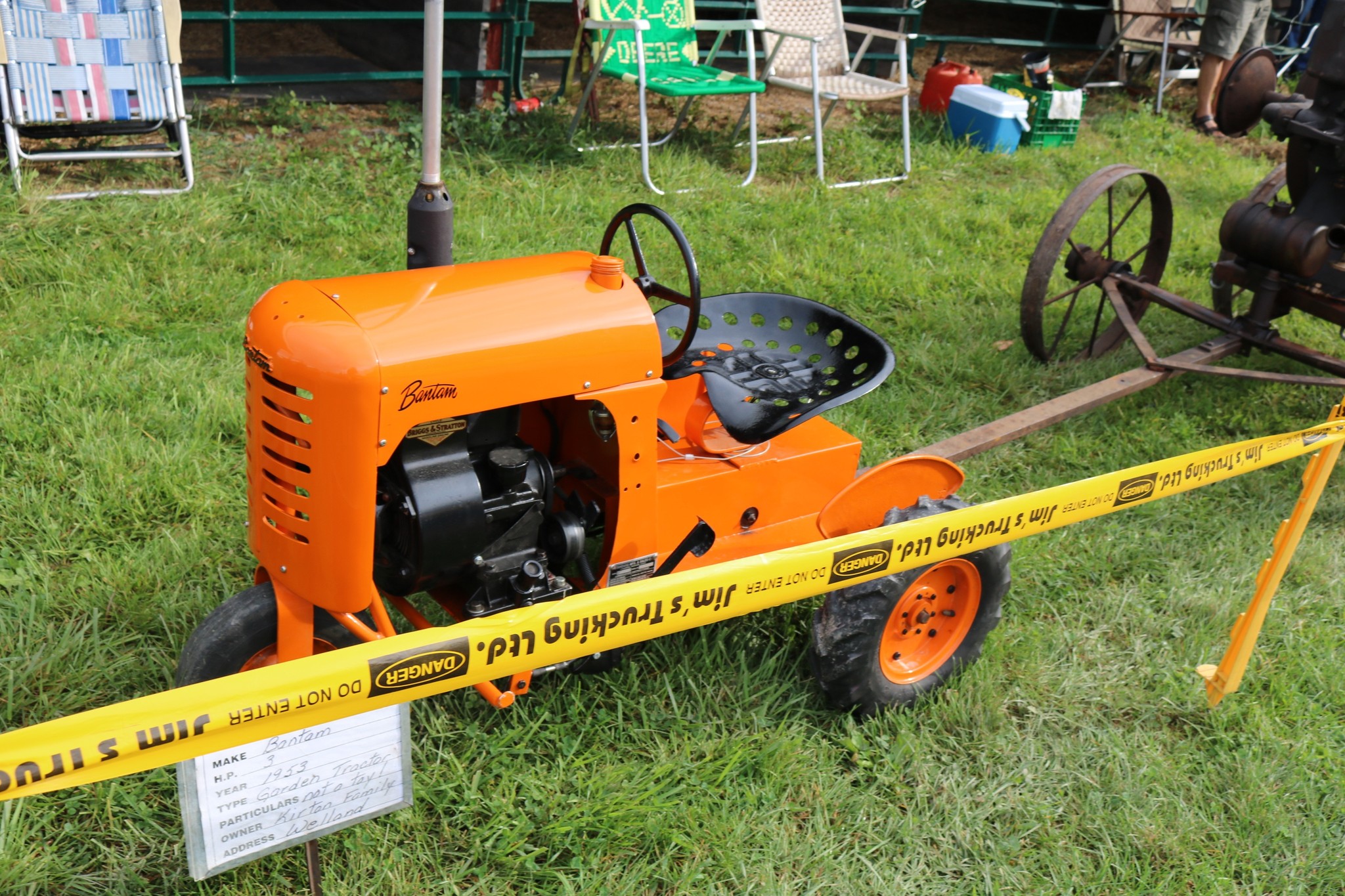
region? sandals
[1192,110,1221,138]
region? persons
[1191,0,1272,139]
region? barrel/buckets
[919,59,983,115]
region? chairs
[731,0,911,187]
[0,0,195,201]
[1082,0,1320,117]
[568,0,771,195]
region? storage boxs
[990,72,1088,150]
[946,84,1031,156]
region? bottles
[509,96,541,117]
